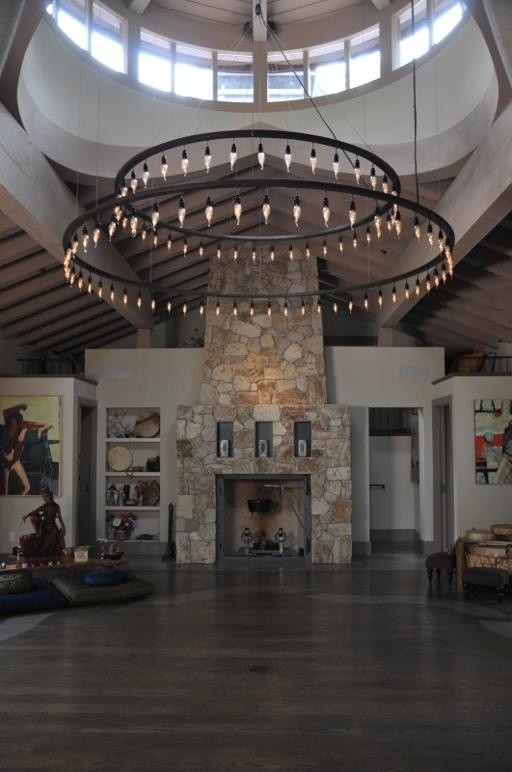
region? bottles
[137,487,143,506]
[146,456,160,471]
[123,485,130,506]
[15,547,23,569]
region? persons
[1,403,54,496]
[17,486,71,559]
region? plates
[129,466,144,471]
[108,446,132,471]
[136,411,160,438]
[143,482,160,506]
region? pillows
[86,570,127,586]
[0,577,67,616]
[0,572,34,595]
[48,568,155,608]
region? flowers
[106,512,138,530]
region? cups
[220,440,229,457]
[259,440,267,456]
[298,439,307,456]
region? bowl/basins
[104,551,125,560]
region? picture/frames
[0,393,62,497]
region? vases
[114,529,129,540]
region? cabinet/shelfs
[105,408,160,544]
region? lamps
[62,2,454,319]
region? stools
[425,553,456,584]
[464,566,509,603]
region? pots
[247,499,272,512]
